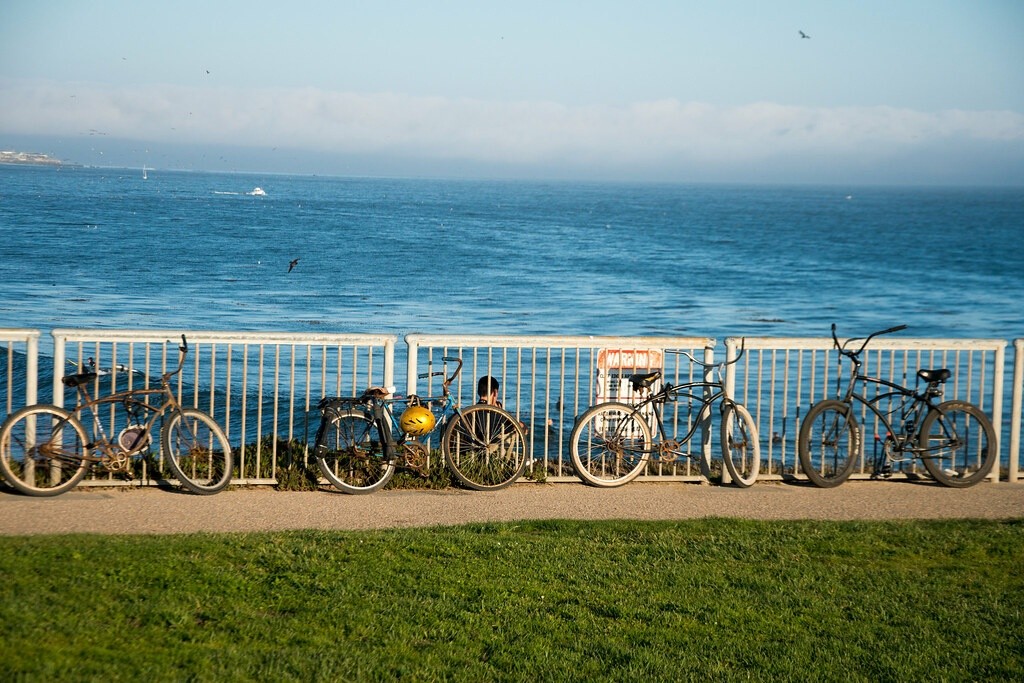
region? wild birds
[799,31,811,38]
[288,258,300,273]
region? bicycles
[0,333,235,498]
[567,337,762,489]
[797,322,998,489]
[310,355,530,495]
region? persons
[455,375,528,454]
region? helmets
[400,406,435,436]
[118,424,151,456]
